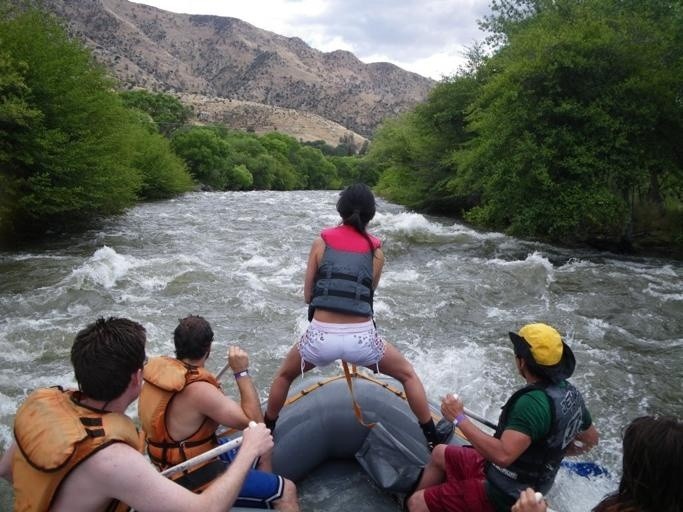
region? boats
[216,372,557,512]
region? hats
[508,322,575,385]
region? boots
[418,417,455,452]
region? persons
[406,324,599,512]
[263,183,454,454]
[510,415,683,512]
[0,317,275,512]
[138,314,301,512]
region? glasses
[618,423,630,441]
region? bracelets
[452,414,467,427]
[234,369,249,378]
